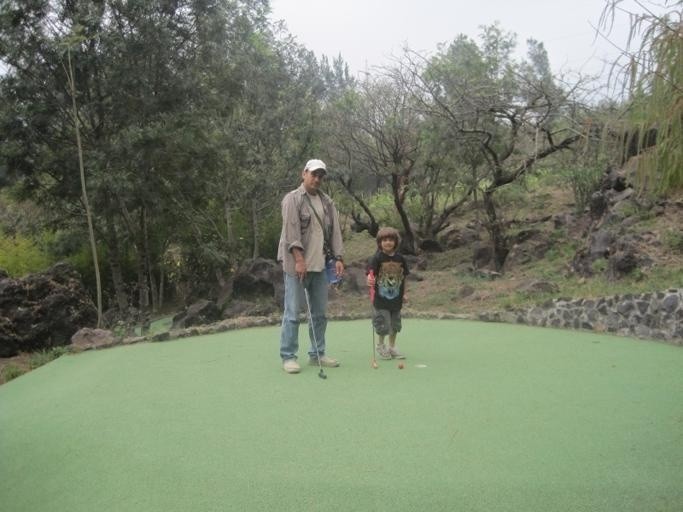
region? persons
[277,157,344,374]
[364,227,409,361]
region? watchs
[335,255,343,262]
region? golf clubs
[302,279,327,379]
[369,269,378,368]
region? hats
[304,159,327,176]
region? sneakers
[376,343,404,359]
[283,359,301,373]
[310,356,339,367]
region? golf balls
[398,363,405,369]
